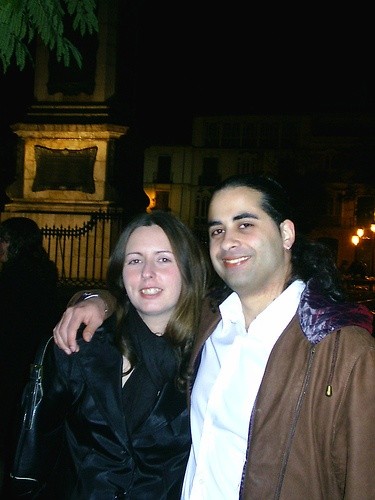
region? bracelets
[80,291,100,300]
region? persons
[4,211,217,500]
[337,258,370,277]
[53,174,375,500]
[0,217,58,472]
